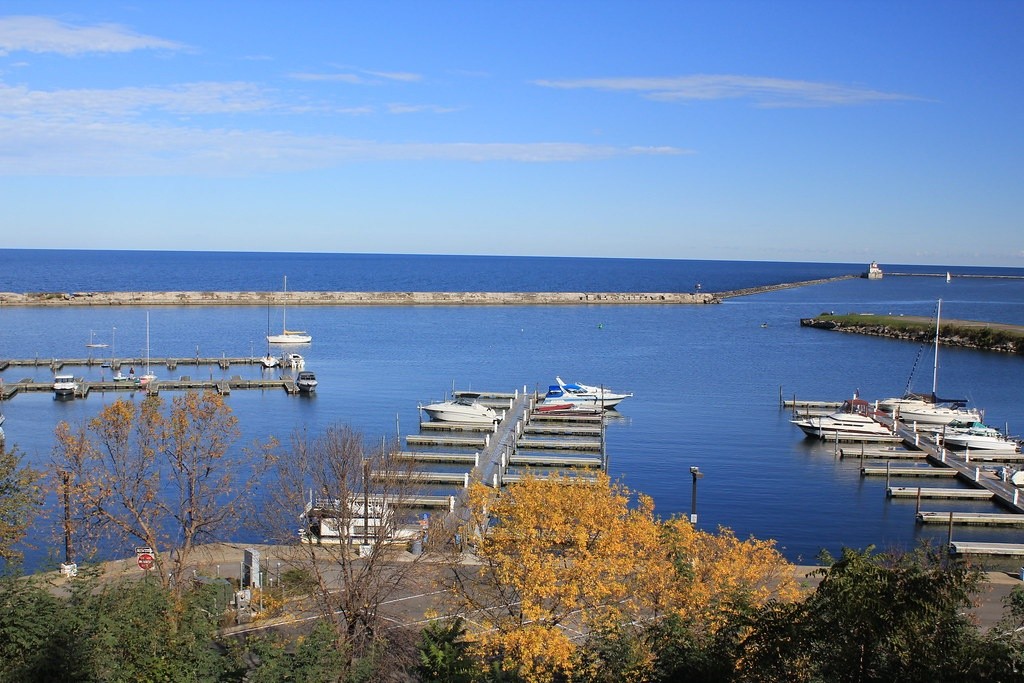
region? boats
[286,352,306,367]
[53,374,78,397]
[537,376,634,416]
[788,413,896,437]
[113,376,137,382]
[300,500,423,548]
[102,362,113,368]
[875,298,1020,453]
[417,400,499,424]
[296,371,319,392]
[1012,470,1024,488]
[146,383,160,396]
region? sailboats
[944,271,952,283]
[137,311,159,384]
[84,329,112,349]
[261,296,280,366]
[266,275,313,343]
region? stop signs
[138,554,154,569]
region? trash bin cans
[412,541,422,555]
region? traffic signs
[135,547,154,554]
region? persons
[129,368,134,380]
[854,389,859,400]
